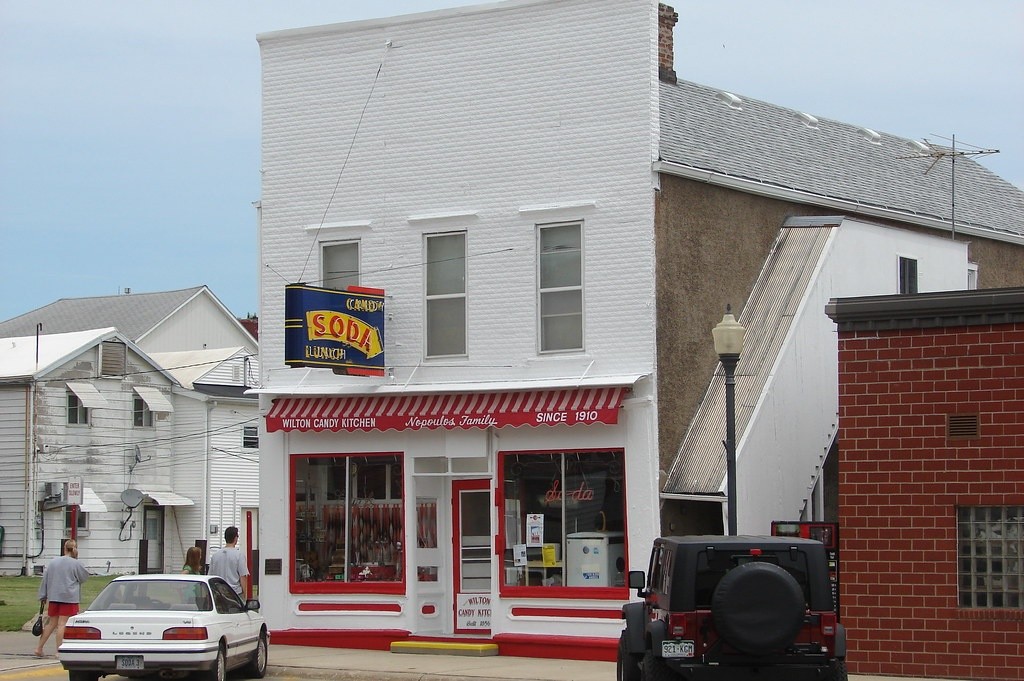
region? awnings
[130,383,175,415]
[73,485,108,517]
[265,387,627,433]
[66,380,110,411]
[141,491,195,509]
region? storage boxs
[543,542,561,562]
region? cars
[57,574,272,681]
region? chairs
[108,602,137,609]
[134,595,153,609]
[170,603,199,611]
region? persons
[208,525,251,606]
[32,538,89,658]
[180,546,202,576]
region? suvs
[617,534,849,680]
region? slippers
[34,651,49,658]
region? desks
[525,560,563,586]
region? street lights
[713,302,746,536]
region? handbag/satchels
[32,600,44,636]
